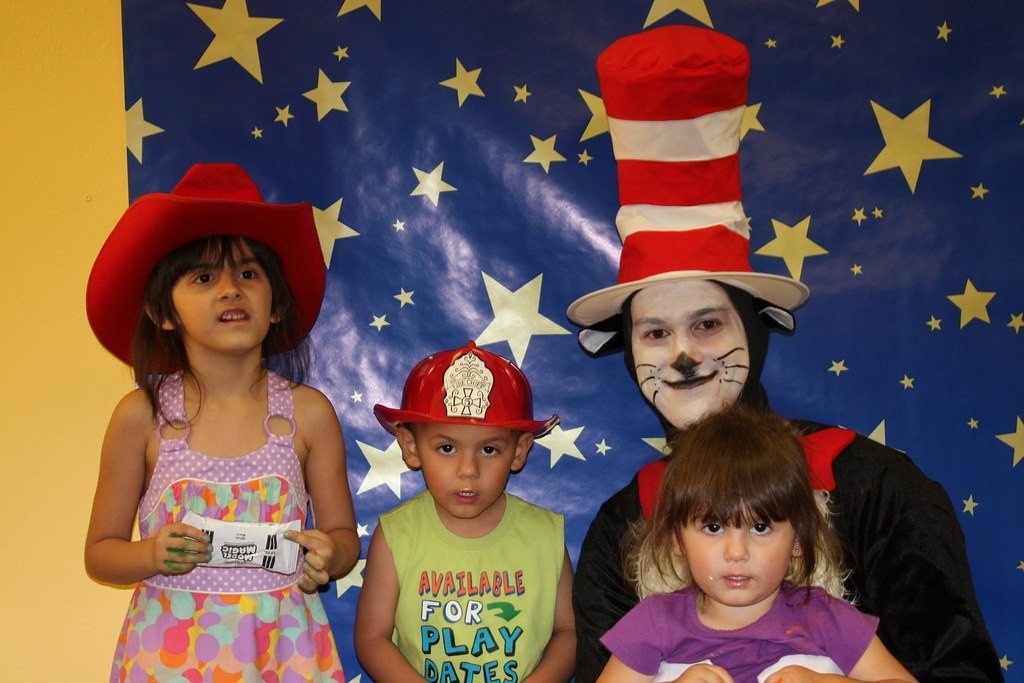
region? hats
[87,162,327,371]
[373,340,559,439]
[566,25,809,316]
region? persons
[560,25,1008,683]
[83,160,363,683]
[594,410,921,682]
[352,349,578,682]
